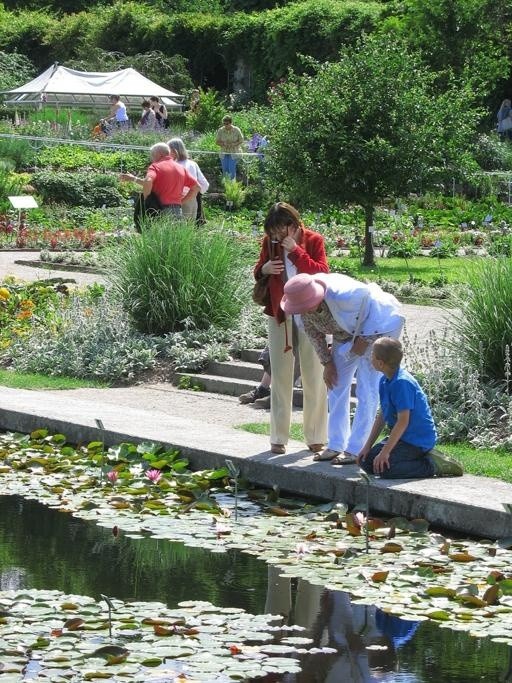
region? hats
[274,270,331,315]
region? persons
[214,115,244,184]
[237,338,301,408]
[251,200,330,456]
[149,96,168,130]
[115,137,210,223]
[137,99,156,131]
[101,94,129,128]
[355,337,465,479]
[190,88,200,110]
[496,98,512,141]
[141,142,203,221]
[279,270,407,464]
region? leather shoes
[270,444,288,454]
[308,444,327,451]
[313,447,342,460]
[333,452,359,465]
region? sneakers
[253,393,271,408]
[430,447,466,476]
[238,384,270,404]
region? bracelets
[134,177,138,183]
[223,142,224,146]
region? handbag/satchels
[251,277,271,306]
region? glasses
[269,225,290,244]
